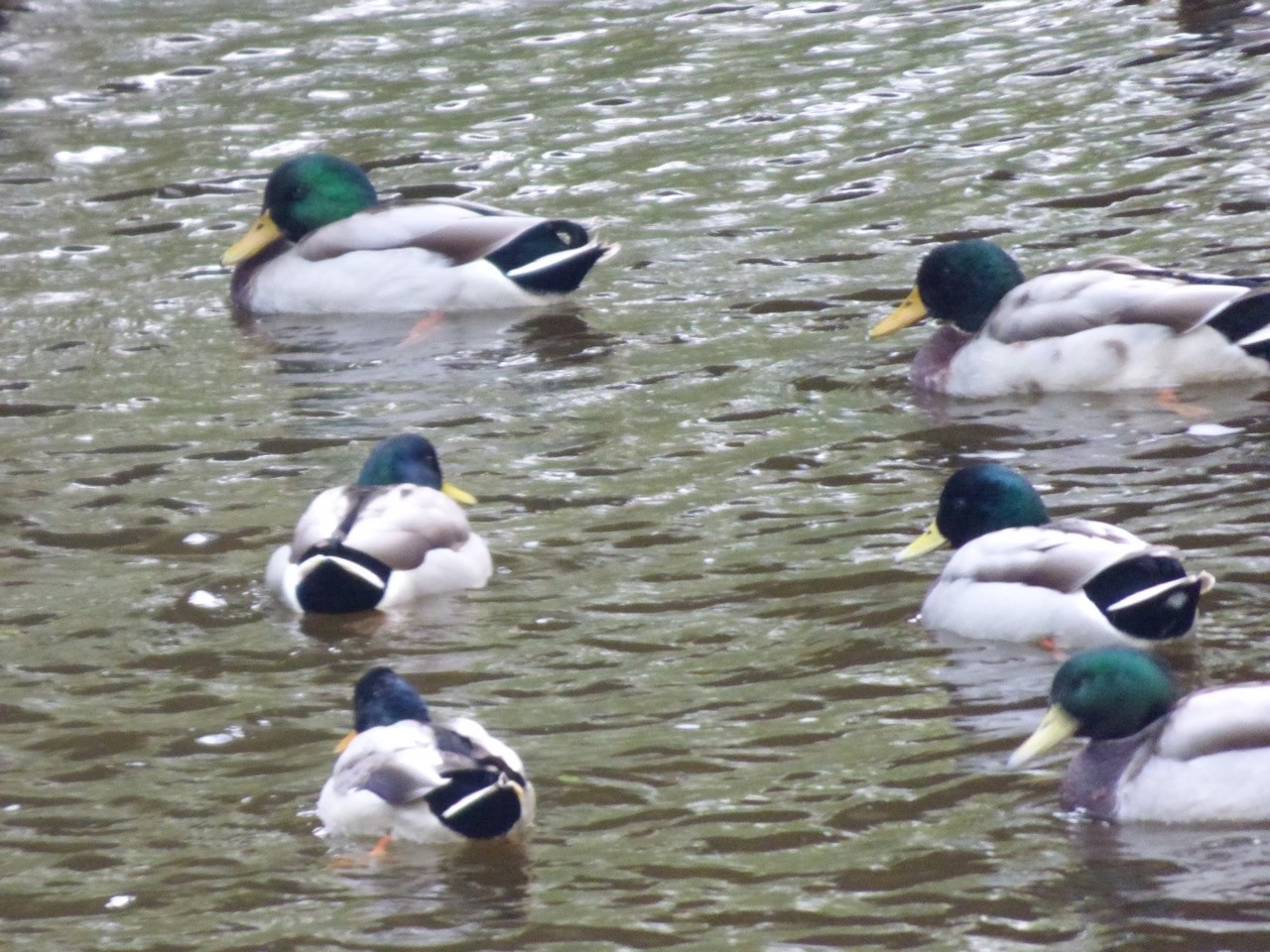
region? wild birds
[220,155,623,316]
[266,433,491,611]
[1007,649,1270,824]
[866,238,1270,397]
[896,465,1215,654]
[316,668,535,842]
